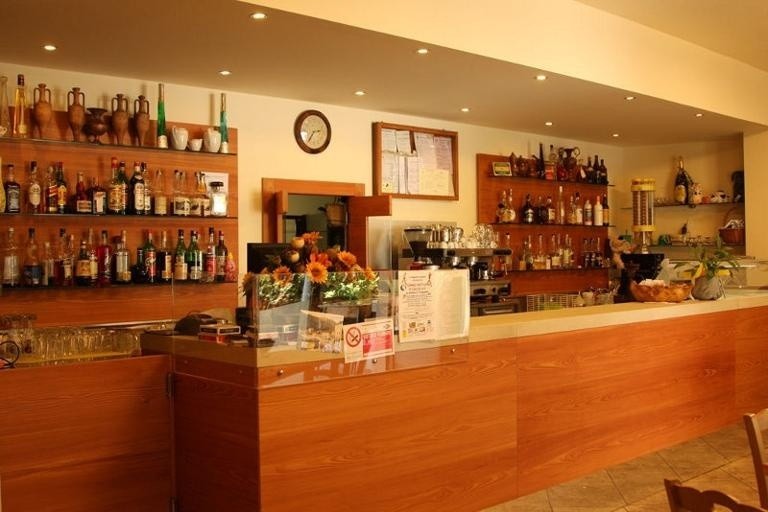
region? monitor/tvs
[248,243,313,274]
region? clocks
[294,108,332,154]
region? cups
[27,324,141,366]
[407,223,496,249]
[186,138,204,152]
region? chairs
[741,408,768,511]
[663,476,768,511]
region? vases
[245,277,376,341]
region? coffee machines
[401,247,513,303]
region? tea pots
[170,125,188,151]
[204,126,222,154]
[562,146,580,181]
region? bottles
[13,73,30,138]
[539,142,544,179]
[492,230,603,271]
[157,82,169,149]
[29,81,151,148]
[673,159,688,205]
[550,142,568,181]
[576,152,608,184]
[3,225,227,286]
[0,154,228,218]
[220,93,229,153]
[498,186,610,226]
[0,312,34,354]
[0,76,12,138]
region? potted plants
[686,237,741,301]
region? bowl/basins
[581,292,593,298]
[630,282,693,302]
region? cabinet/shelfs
[476,153,616,305]
[0,102,238,510]
[621,199,745,250]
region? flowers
[239,231,382,301]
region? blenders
[404,229,439,270]
